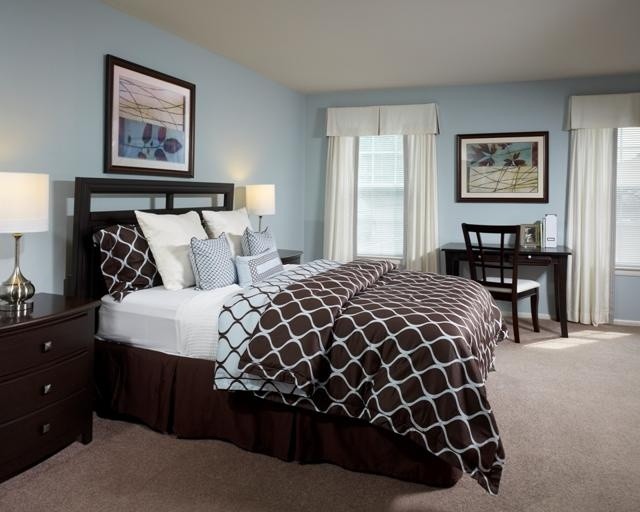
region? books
[534,218,547,248]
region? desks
[441,242,572,338]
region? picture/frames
[520,224,540,247]
[455,131,549,203]
[103,53,196,179]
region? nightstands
[277,249,303,264]
[0,293,102,485]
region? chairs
[462,223,540,343]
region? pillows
[202,206,254,255]
[235,246,284,288]
[135,210,208,291]
[189,231,238,291]
[240,226,275,256]
[92,223,163,304]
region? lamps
[1,172,50,313]
[247,184,276,231]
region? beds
[72,176,510,498]
